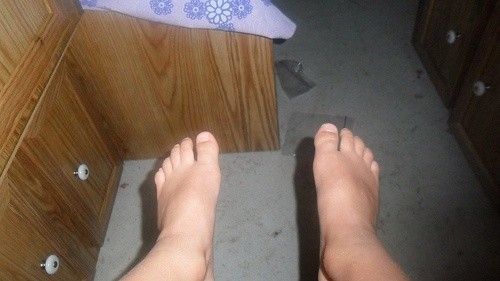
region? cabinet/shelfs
[411,0,500,208]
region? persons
[119,123,412,281]
